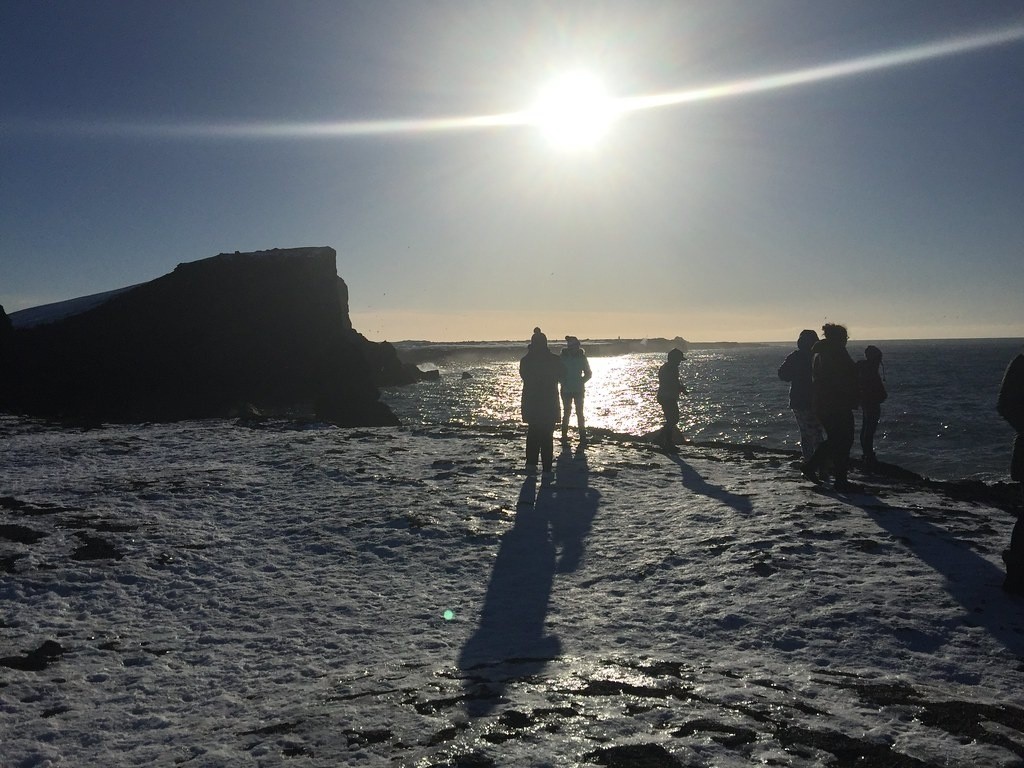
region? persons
[519,327,593,478]
[997,352,1024,604]
[657,348,688,455]
[777,324,888,493]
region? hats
[668,348,687,361]
[565,336,579,347]
[865,345,882,361]
[531,327,548,347]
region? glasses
[846,336,849,339]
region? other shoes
[666,441,680,449]
[653,436,665,448]
[801,469,821,484]
[526,464,538,471]
[542,472,552,479]
[1002,577,1024,593]
